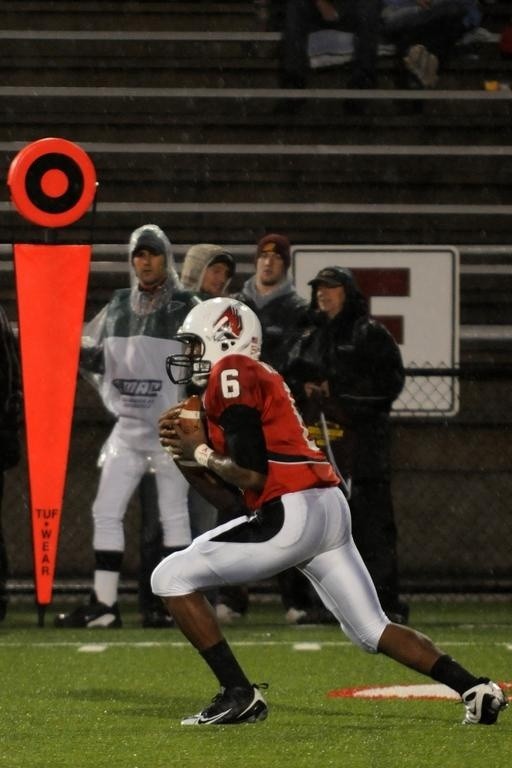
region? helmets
[165,296,264,388]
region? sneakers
[179,682,270,727]
[53,588,123,629]
[454,676,509,726]
[214,604,245,626]
[286,607,309,623]
[141,598,174,629]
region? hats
[131,229,165,260]
[257,234,291,269]
[307,267,351,289]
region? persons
[181,244,236,538]
[150,298,507,726]
[0,303,28,619]
[52,223,190,630]
[289,267,409,622]
[269,0,512,116]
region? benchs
[1,0,512,350]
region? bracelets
[193,444,214,468]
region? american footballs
[178,394,203,434]
